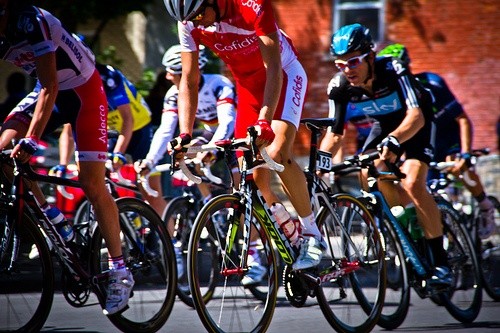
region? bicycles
[0,116,500,333]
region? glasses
[334,52,370,70]
[165,66,183,74]
[191,0,209,21]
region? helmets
[163,0,205,25]
[76,34,84,42]
[330,24,372,56]
[161,44,209,70]
[376,43,410,62]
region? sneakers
[103,270,134,315]
[176,246,185,279]
[241,244,266,287]
[424,266,455,289]
[292,232,327,270]
[478,202,494,239]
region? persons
[48,34,184,280]
[132,44,267,286]
[0,0,136,316]
[304,23,455,297]
[164,0,328,270]
[357,43,495,250]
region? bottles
[405,202,425,237]
[40,201,76,243]
[128,211,141,230]
[389,204,418,243]
[270,202,298,242]
[452,201,472,229]
